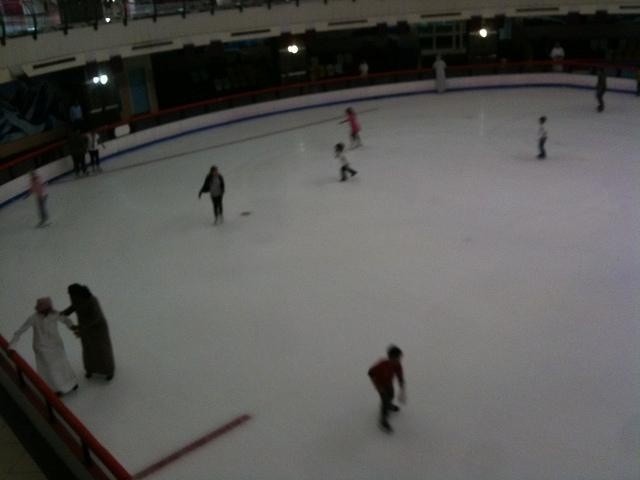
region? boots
[380,405,400,432]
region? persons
[596,69,606,113]
[551,43,565,79]
[537,116,548,158]
[199,165,224,225]
[23,171,49,228]
[369,347,404,432]
[335,107,362,182]
[10,283,115,398]
[68,101,105,178]
[433,56,447,94]
